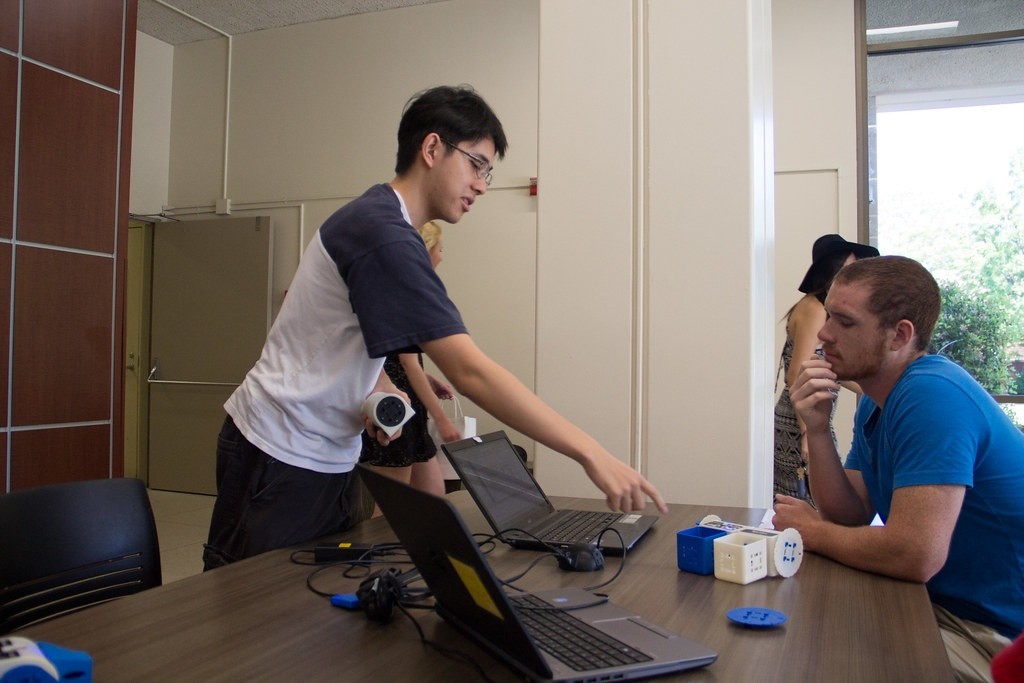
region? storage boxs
[714,531,768,585]
[676,526,727,577]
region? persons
[360,220,461,518]
[205,86,668,570]
[773,234,880,512]
[771,256,1023,683]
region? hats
[797,233,880,293]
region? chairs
[0,476,164,638]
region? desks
[0,489,954,683]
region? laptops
[440,430,660,551]
[355,463,718,683]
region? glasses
[440,137,493,188]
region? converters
[314,543,374,564]
